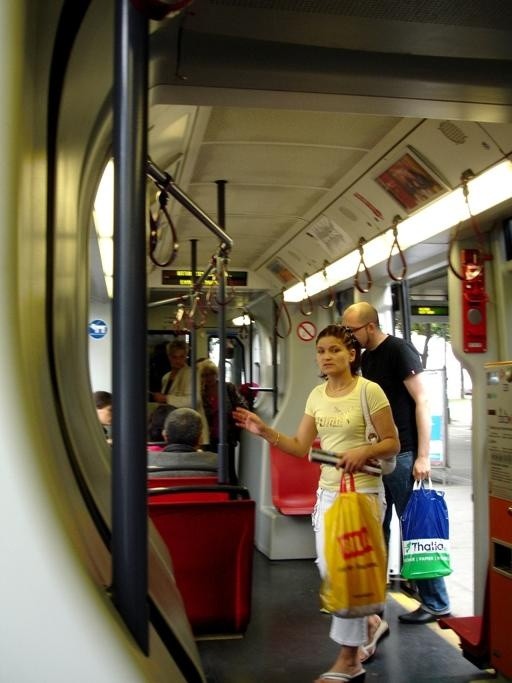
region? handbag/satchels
[320,471,386,618]
[361,380,396,475]
[400,478,452,580]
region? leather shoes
[399,607,451,623]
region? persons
[148,406,219,475]
[92,390,114,447]
[340,301,452,625]
[149,340,172,402]
[150,339,209,451]
[199,356,247,483]
[229,325,393,681]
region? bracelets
[270,432,280,446]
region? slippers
[319,667,366,682]
[362,621,390,663]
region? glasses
[351,323,368,334]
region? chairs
[438,560,489,669]
[145,440,256,640]
[270,439,321,516]
